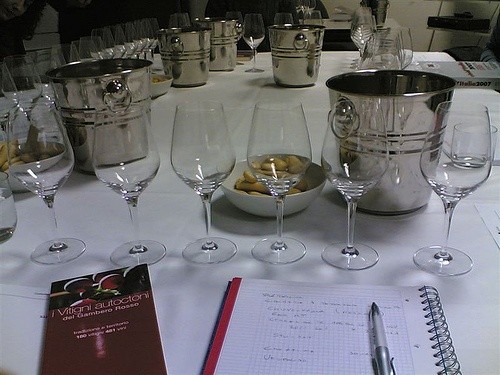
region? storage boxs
[453,61,500,90]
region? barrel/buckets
[157,26,213,87]
[323,69,458,216]
[193,15,238,71]
[43,57,153,177]
[268,24,327,87]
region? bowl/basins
[151,74,174,97]
[217,153,327,218]
[2,138,67,193]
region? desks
[0,51,500,375]
[301,17,400,52]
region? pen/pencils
[372,301,396,375]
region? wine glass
[91,103,168,269]
[320,99,389,270]
[6,100,87,265]
[0,14,191,144]
[241,14,266,73]
[413,101,492,277]
[246,98,313,264]
[170,101,238,265]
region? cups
[225,11,244,41]
[0,171,18,245]
[290,0,317,25]
[450,122,499,171]
[350,7,413,70]
[303,10,323,26]
[274,13,294,25]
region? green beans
[0,140,38,172]
[151,75,168,83]
[234,155,307,196]
